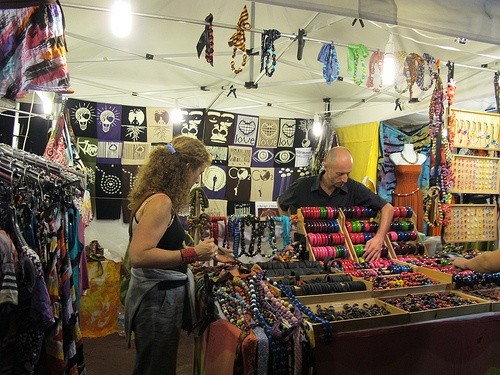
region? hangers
[0,142,90,260]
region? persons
[447,248,500,273]
[390,144,427,231]
[126,136,237,375]
[280,146,394,262]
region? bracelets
[257,206,426,296]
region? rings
[446,157,498,239]
[213,252,218,256]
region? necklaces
[454,119,500,149]
[191,211,298,330]
[401,150,419,165]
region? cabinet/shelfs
[82,256,121,336]
[193,253,500,374]
